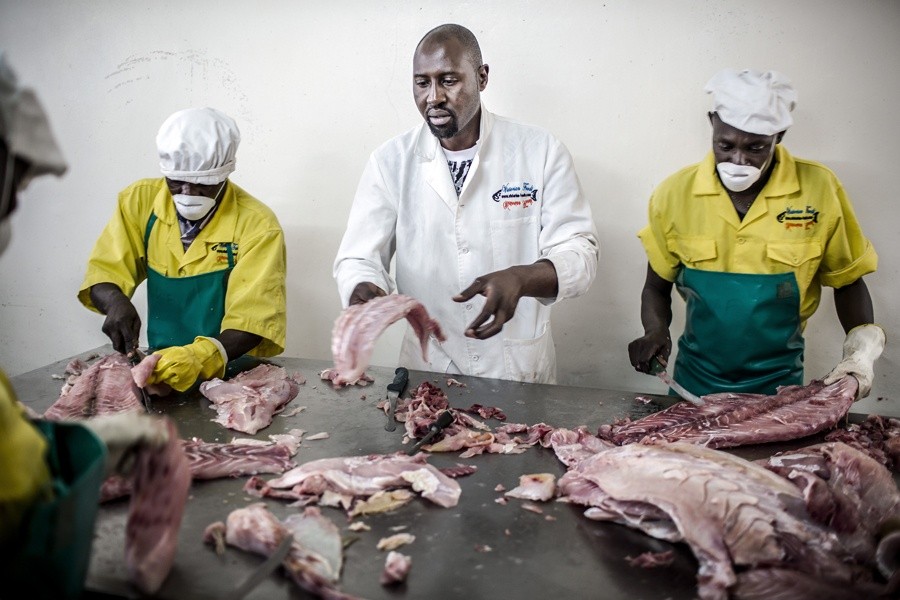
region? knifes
[651,357,705,407]
[386,367,409,431]
[126,350,151,412]
[223,533,293,599]
[409,409,455,455]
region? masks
[173,181,226,220]
[717,136,776,192]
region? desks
[13,341,900,600]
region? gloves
[80,415,167,475]
[147,335,228,392]
[824,324,886,401]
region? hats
[706,68,796,137]
[156,108,240,185]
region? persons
[76,108,288,396]
[332,23,599,384]
[625,67,887,402]
[0,51,172,600]
